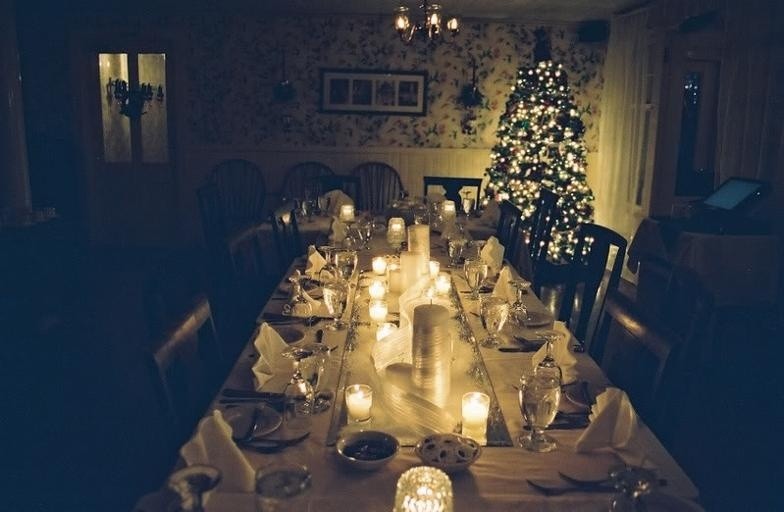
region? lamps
[106,78,164,120]
[393,0,463,51]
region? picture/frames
[319,68,427,117]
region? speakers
[578,19,610,43]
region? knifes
[562,382,618,431]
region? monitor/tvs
[695,177,773,218]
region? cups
[344,384,372,427]
[461,391,490,446]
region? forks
[520,466,668,503]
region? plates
[164,201,374,512]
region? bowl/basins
[335,430,400,472]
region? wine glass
[361,194,585,367]
[517,371,562,453]
